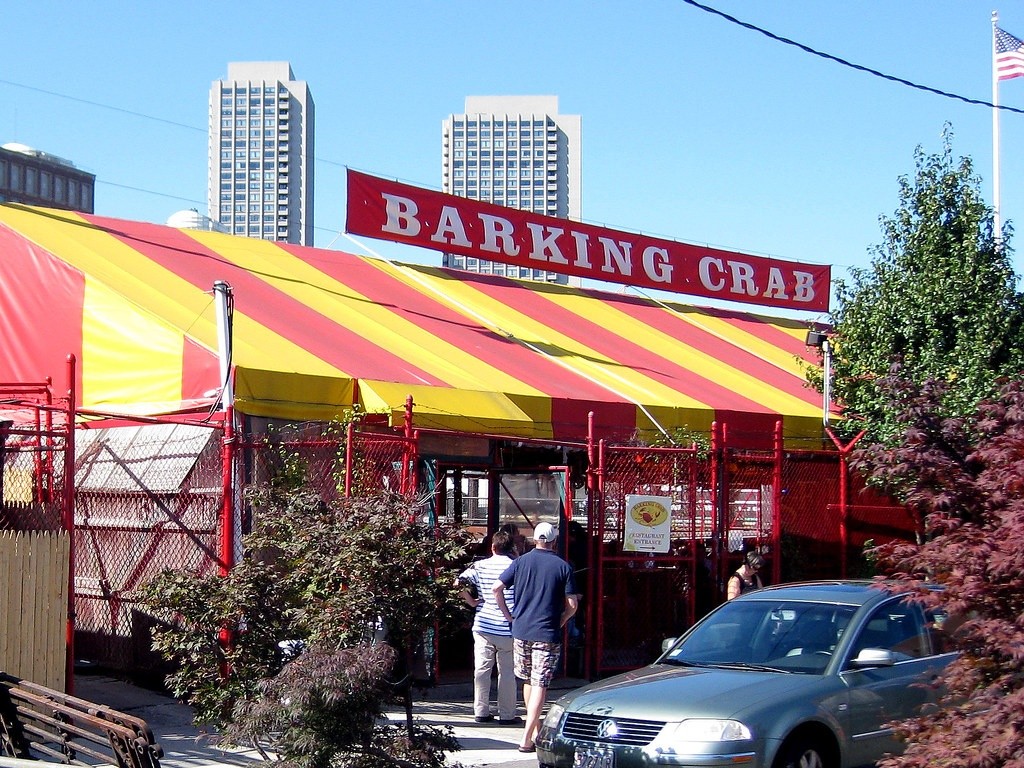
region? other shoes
[499,717,524,725]
[476,713,494,722]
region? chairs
[883,617,912,650]
[782,620,834,658]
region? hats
[534,522,559,542]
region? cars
[533,577,982,768]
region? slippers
[519,742,536,752]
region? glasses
[752,565,759,572]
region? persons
[452,531,522,725]
[727,551,763,601]
[491,522,578,753]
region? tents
[0,200,866,450]
